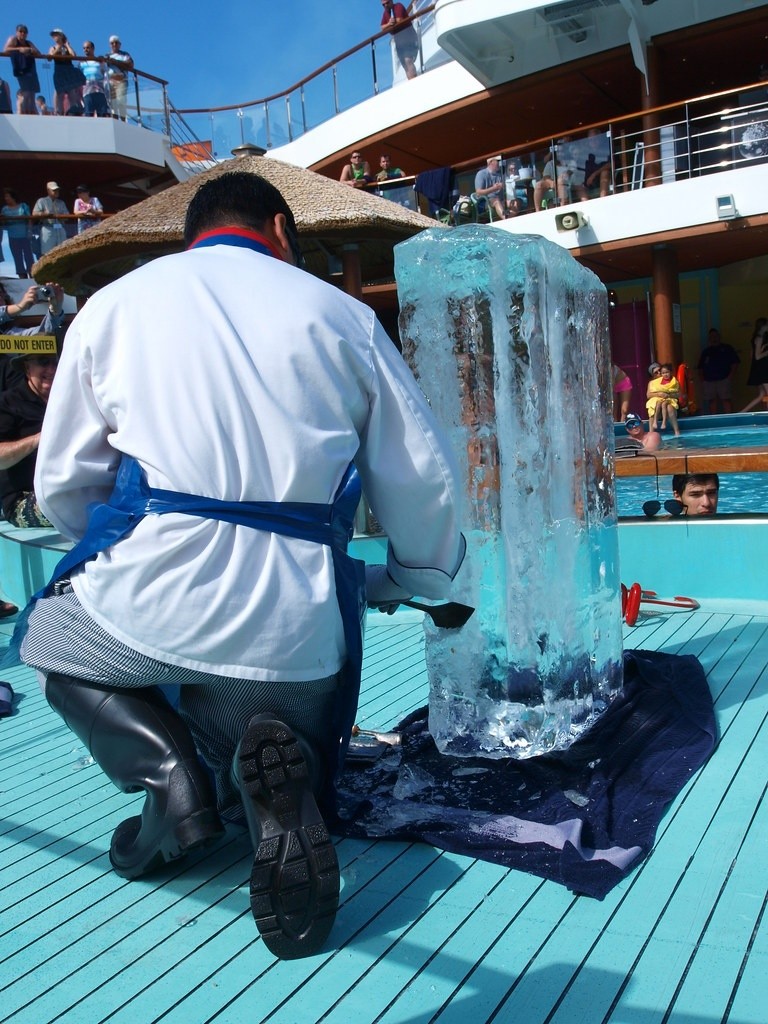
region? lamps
[716,194,739,221]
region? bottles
[391,8,395,26]
[527,163,530,168]
[521,164,523,169]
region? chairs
[456,193,498,223]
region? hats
[76,186,87,193]
[9,353,35,370]
[624,414,641,425]
[47,182,59,191]
[648,364,660,375]
[51,29,64,36]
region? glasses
[25,357,61,368]
[626,421,640,429]
[642,500,688,517]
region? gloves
[363,561,414,614]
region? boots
[44,666,225,882]
[230,714,340,960]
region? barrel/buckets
[518,168,532,179]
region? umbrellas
[29,144,453,300]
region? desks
[515,178,537,215]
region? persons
[48,29,135,122]
[381,0,418,79]
[534,129,611,211]
[15,171,466,960]
[4,24,54,115]
[611,362,632,421]
[32,181,69,255]
[741,317,768,412]
[340,150,373,192]
[624,412,661,450]
[474,155,529,219]
[0,78,12,113]
[646,363,683,434]
[0,190,33,278]
[0,281,65,616]
[375,154,407,206]
[672,472,719,515]
[698,328,740,414]
[74,184,103,234]
